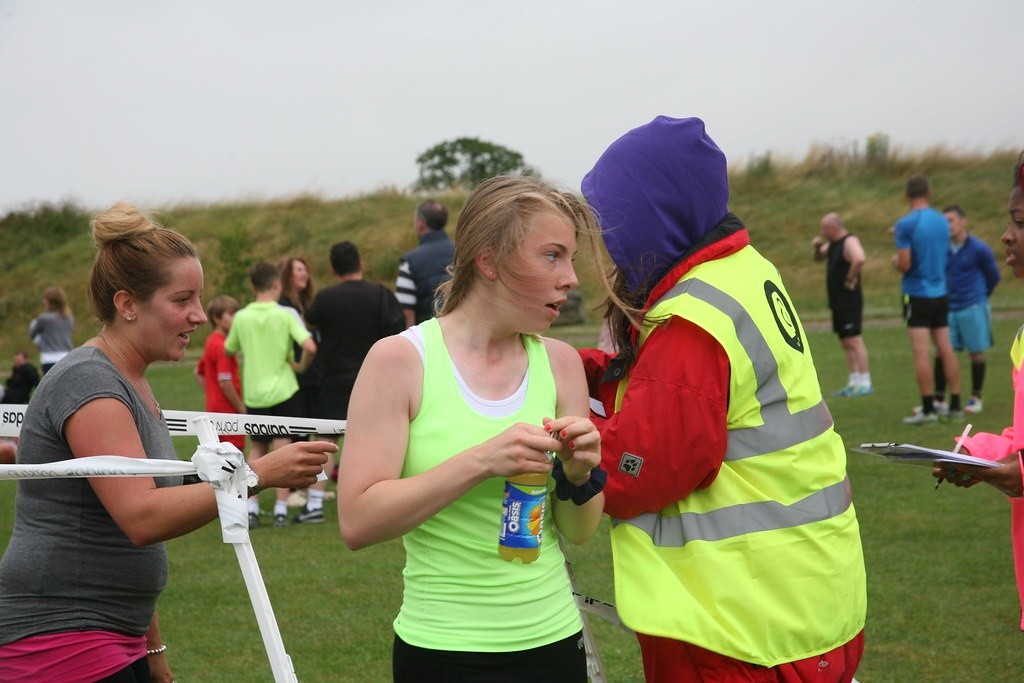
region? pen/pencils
[935,423,973,489]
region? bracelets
[553,455,607,506]
[147,645,167,655]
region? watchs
[244,463,260,496]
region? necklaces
[97,333,161,414]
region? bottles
[498,450,552,565]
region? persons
[887,176,1003,426]
[1,203,339,682]
[336,174,606,683]
[394,199,456,330]
[932,148,1024,633]
[574,115,868,683]
[811,211,877,399]
[1,286,75,464]
[193,240,406,531]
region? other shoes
[938,413,963,423]
[847,385,873,396]
[912,399,947,414]
[248,512,262,529]
[902,411,938,427]
[831,385,853,397]
[964,396,982,414]
[292,504,325,524]
[272,511,288,527]
